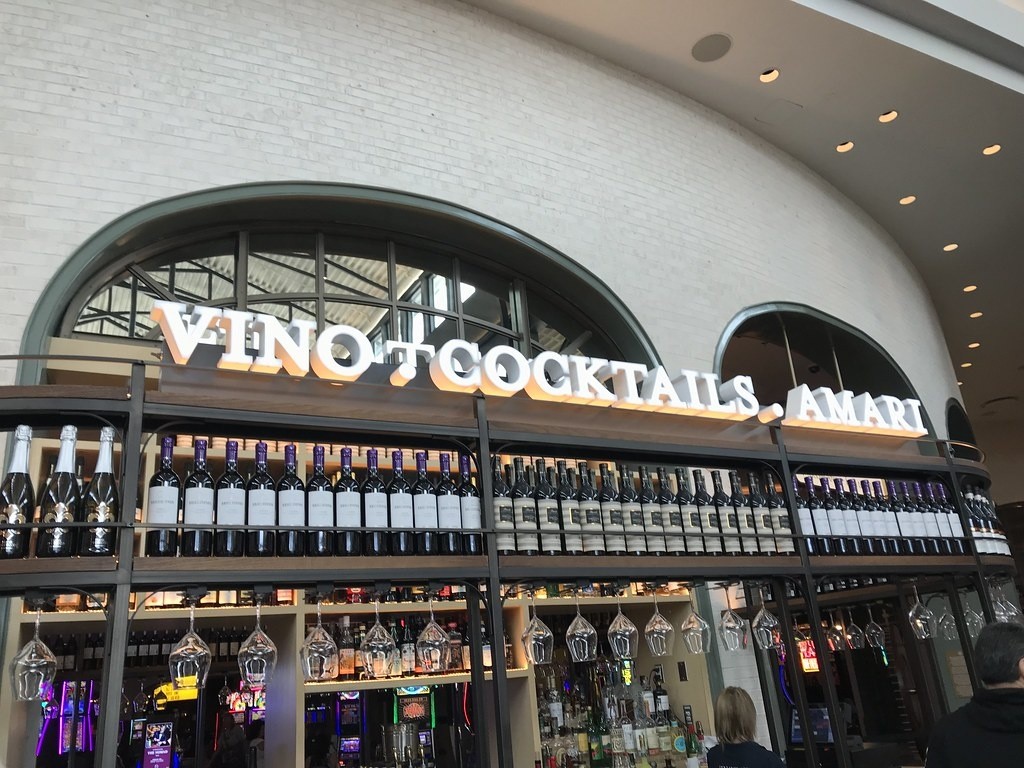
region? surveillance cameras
[808,363,820,373]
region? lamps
[799,631,820,672]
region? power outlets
[654,664,666,683]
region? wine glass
[360,592,396,675]
[604,584,639,660]
[238,592,279,686]
[417,593,452,672]
[9,592,57,702]
[300,591,338,681]
[120,678,167,713]
[169,592,211,690]
[519,585,553,665]
[217,673,232,706]
[563,585,597,662]
[907,579,1024,639]
[643,582,782,656]
[789,603,885,651]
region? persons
[147,724,171,747]
[206,713,265,768]
[926,622,1024,768]
[304,727,336,768]
[707,687,785,768]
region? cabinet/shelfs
[0,363,1023,767]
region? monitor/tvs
[787,701,846,746]
[338,735,360,754]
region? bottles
[145,436,484,557]
[531,613,708,768]
[0,424,144,559]
[491,455,797,557]
[304,616,513,683]
[792,474,854,556]
[42,626,269,671]
[960,483,1012,556]
[847,479,968,556]
